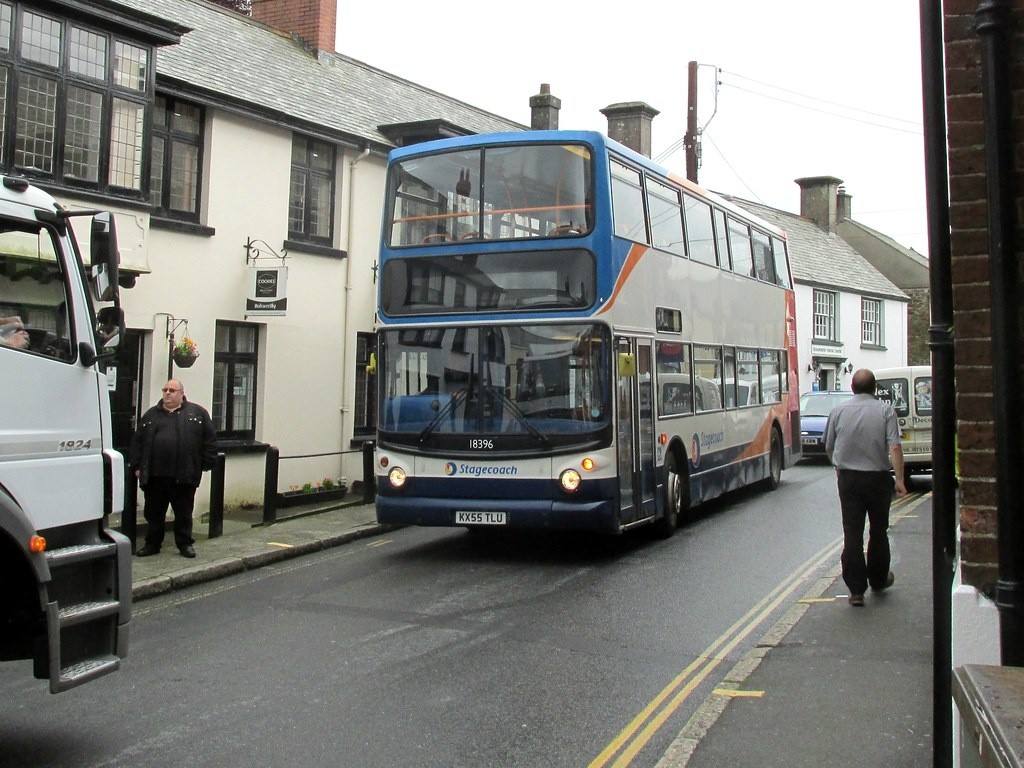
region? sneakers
[137,547,161,557]
[848,593,865,607]
[180,543,196,558]
[871,571,894,593]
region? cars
[797,389,854,460]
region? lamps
[808,360,819,371]
[844,362,853,374]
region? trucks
[1,176,132,693]
[514,344,593,429]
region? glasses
[161,387,182,394]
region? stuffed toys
[915,381,930,406]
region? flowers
[171,337,200,357]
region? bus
[366,129,801,537]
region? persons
[131,380,219,558]
[0,316,29,350]
[98,314,119,339]
[821,369,907,605]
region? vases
[173,356,198,368]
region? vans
[638,373,719,481]
[874,366,933,481]
[716,377,758,408]
